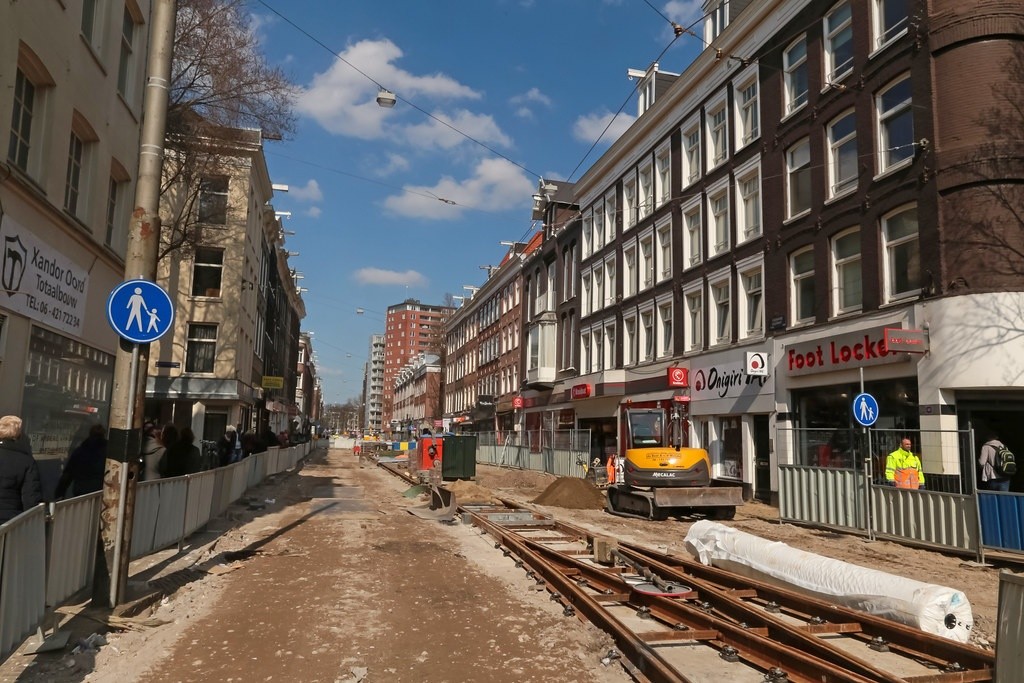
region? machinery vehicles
[605,405,746,522]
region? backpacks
[983,444,1018,478]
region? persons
[885,438,926,489]
[978,433,1010,492]
[268,426,289,448]
[216,423,258,467]
[141,421,201,481]
[0,415,42,526]
[54,424,107,502]
